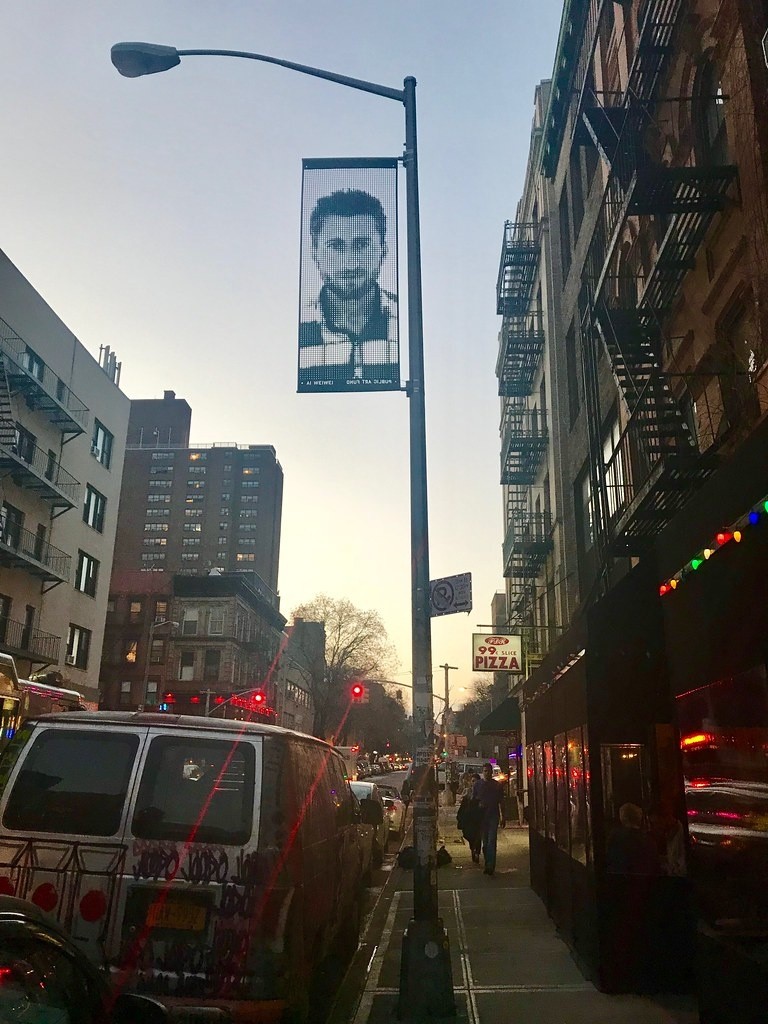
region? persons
[457,773,482,865]
[299,188,399,380]
[471,763,507,876]
[457,768,473,803]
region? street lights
[110,39,464,1024]
[142,618,180,710]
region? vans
[0,711,386,1023]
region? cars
[319,746,413,892]
[0,892,171,1024]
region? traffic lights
[250,693,267,704]
[351,684,369,704]
